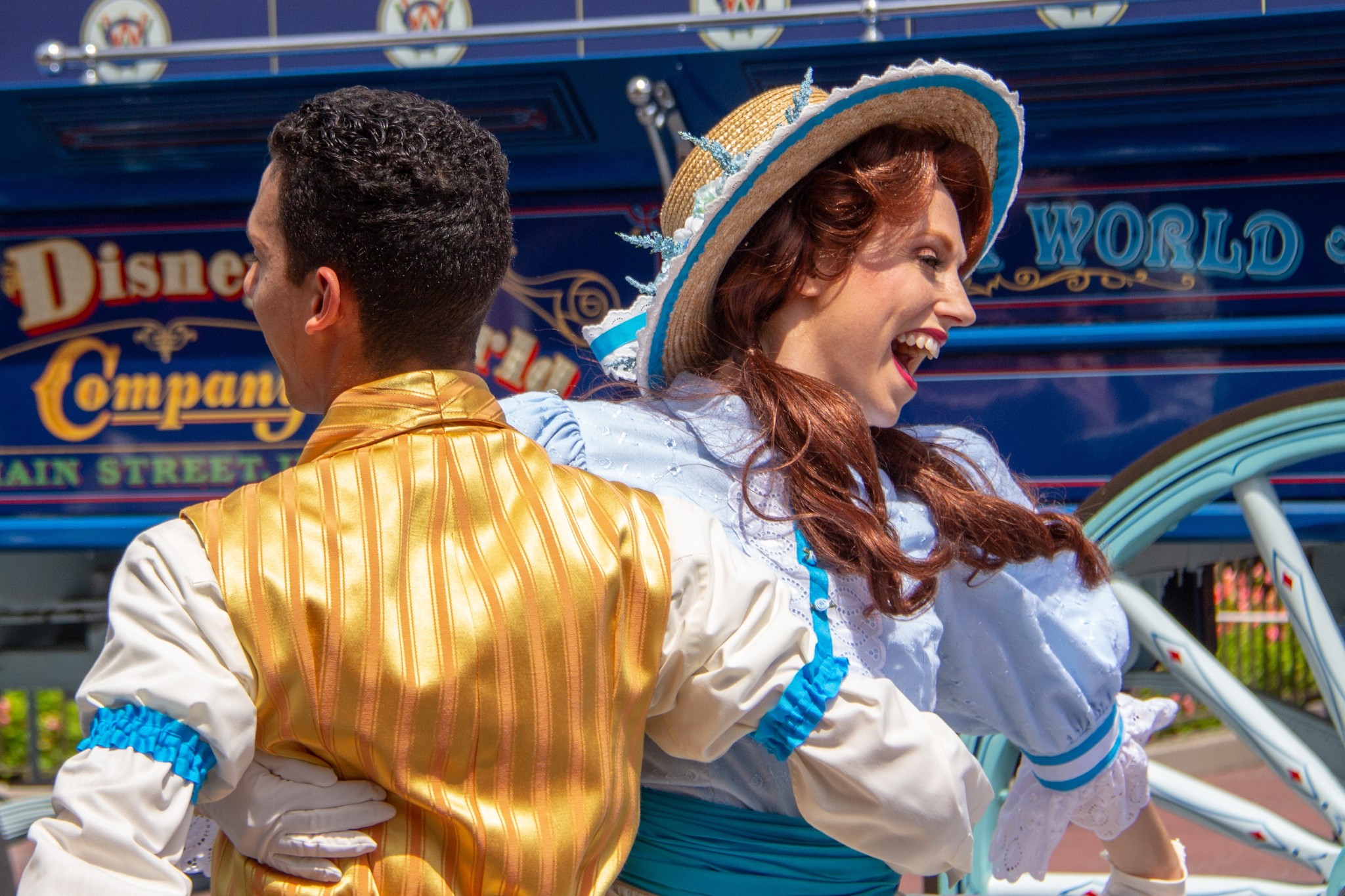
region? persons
[192,59,1188,896]
[14,85,994,896]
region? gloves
[195,750,398,883]
[1101,836,1187,895]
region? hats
[581,57,1028,400]
